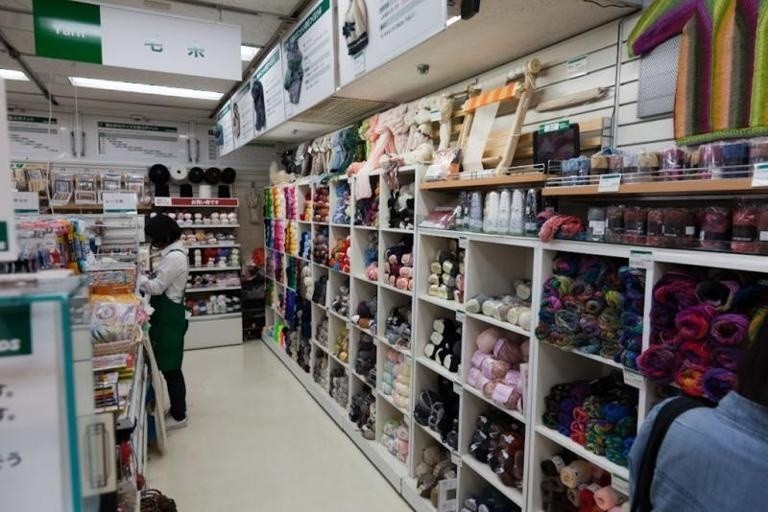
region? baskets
[117,488,178,512]
[91,322,142,357]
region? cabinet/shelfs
[261,164,768,512]
[0,193,245,512]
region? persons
[137,214,191,433]
[625,311,768,512]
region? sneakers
[164,408,189,430]
